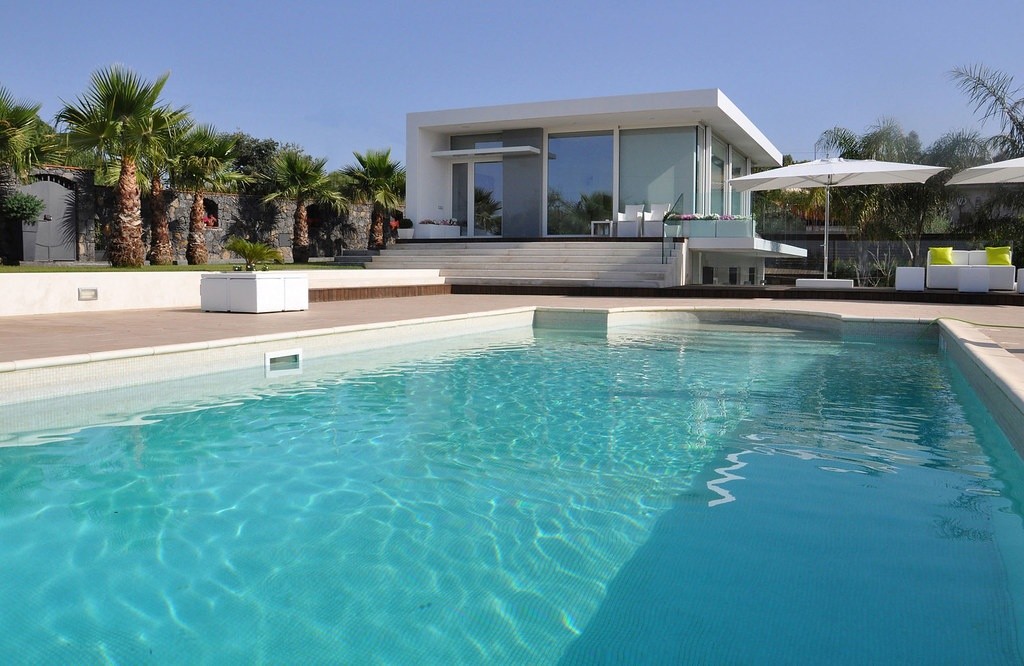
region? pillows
[985,246,1011,266]
[929,247,954,265]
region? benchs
[926,250,1015,290]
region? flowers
[419,217,457,226]
[676,213,757,220]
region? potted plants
[397,219,415,239]
[662,211,682,237]
[200,236,309,314]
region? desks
[591,221,616,237]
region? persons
[205,212,217,227]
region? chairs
[644,204,671,237]
[617,204,645,237]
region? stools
[895,267,925,291]
[959,267,990,292]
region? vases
[418,224,460,237]
[683,221,757,237]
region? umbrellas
[944,158,1024,186]
[729,159,951,279]
